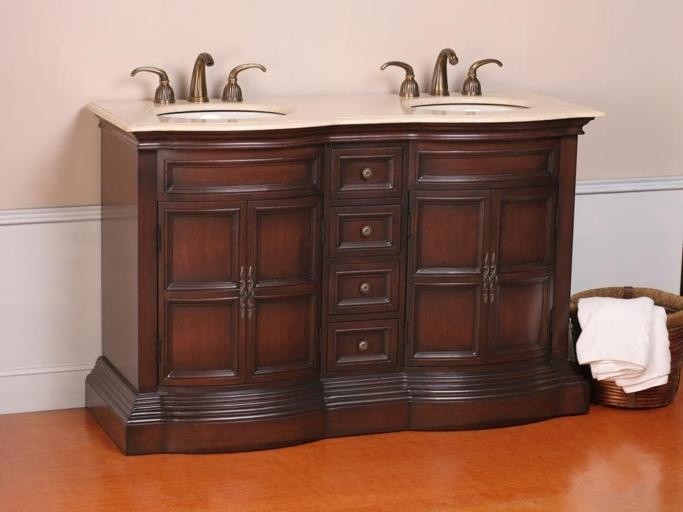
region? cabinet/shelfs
[408,124,590,433]
[321,124,408,438]
[85,113,322,456]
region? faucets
[429,48,459,96]
[187,51,215,102]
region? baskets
[568,287,683,408]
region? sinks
[407,101,533,113]
[152,104,289,121]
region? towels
[574,296,656,380]
[612,306,672,396]
